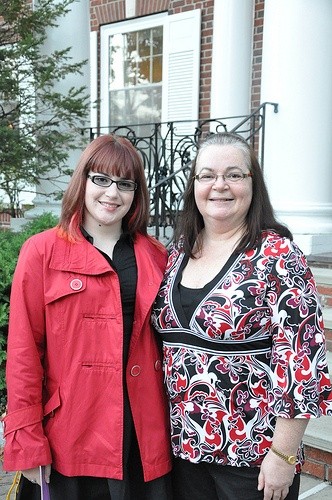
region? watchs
[271,445,297,464]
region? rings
[31,479,37,484]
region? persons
[149,131,332,500]
[2,135,171,500]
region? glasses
[86,174,138,191]
[192,171,254,185]
